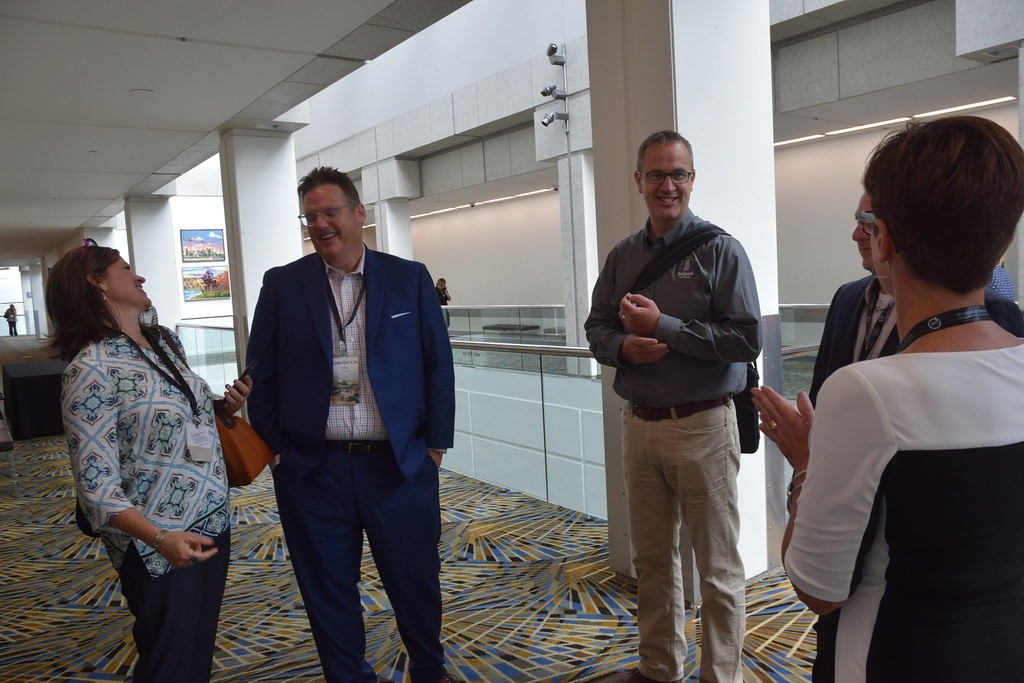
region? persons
[47,245,253,683]
[6,305,18,336]
[242,167,457,683]
[584,131,764,683]
[435,278,451,330]
[753,117,1023,683]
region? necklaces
[897,305,993,354]
[859,280,896,360]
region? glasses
[81,236,98,263]
[298,200,358,228]
[861,221,873,235]
[633,169,695,185]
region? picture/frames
[182,264,232,301]
[180,229,226,262]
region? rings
[239,399,245,403]
[190,552,198,563]
[621,315,625,321]
[770,420,777,429]
[189,562,194,568]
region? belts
[623,397,729,421]
[324,439,390,455]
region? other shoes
[440,673,466,683]
[627,669,684,683]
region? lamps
[542,110,571,127]
[540,84,568,99]
[546,42,565,65]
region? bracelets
[152,529,169,550]
[786,469,805,512]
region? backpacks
[4,309,11,318]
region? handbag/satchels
[212,399,275,487]
[735,358,760,454]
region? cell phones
[221,361,257,406]
